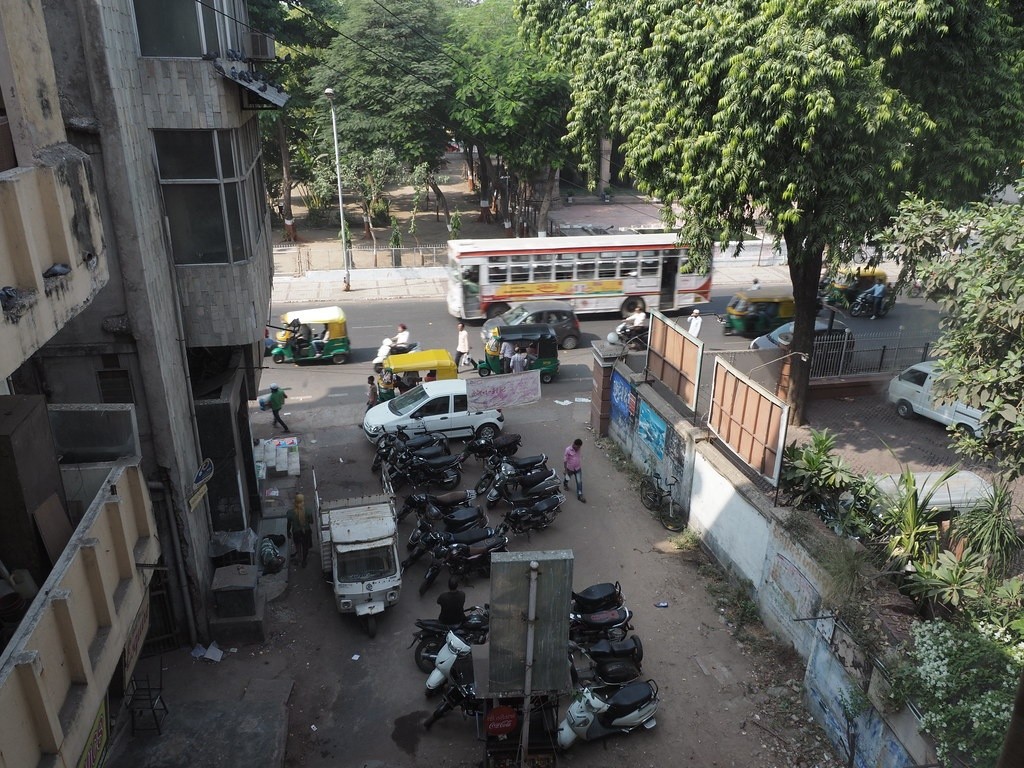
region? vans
[886,361,1010,446]
[874,472,994,543]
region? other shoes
[281,429,290,433]
[270,421,275,428]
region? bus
[444,233,712,320]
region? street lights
[325,89,352,291]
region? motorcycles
[824,266,888,307]
[370,421,659,750]
[309,464,404,636]
[612,321,651,349]
[909,280,927,297]
[720,293,798,339]
[477,324,559,385]
[272,308,353,366]
[849,294,889,317]
[372,349,458,405]
[373,337,422,375]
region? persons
[437,576,466,624]
[621,307,647,343]
[261,383,291,433]
[863,278,885,320]
[286,494,314,568]
[747,278,760,291]
[879,281,893,314]
[389,323,409,354]
[500,341,515,374]
[454,322,478,374]
[294,323,310,355]
[509,347,527,373]
[311,324,329,358]
[686,308,703,338]
[563,438,586,503]
[358,375,378,430]
[258,533,286,574]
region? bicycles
[641,474,687,532]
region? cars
[362,378,503,445]
[481,299,582,350]
[748,316,854,370]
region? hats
[270,383,278,390]
[692,309,700,314]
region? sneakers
[473,364,478,370]
[563,482,569,491]
[870,316,877,320]
[314,353,323,358]
[577,495,586,503]
